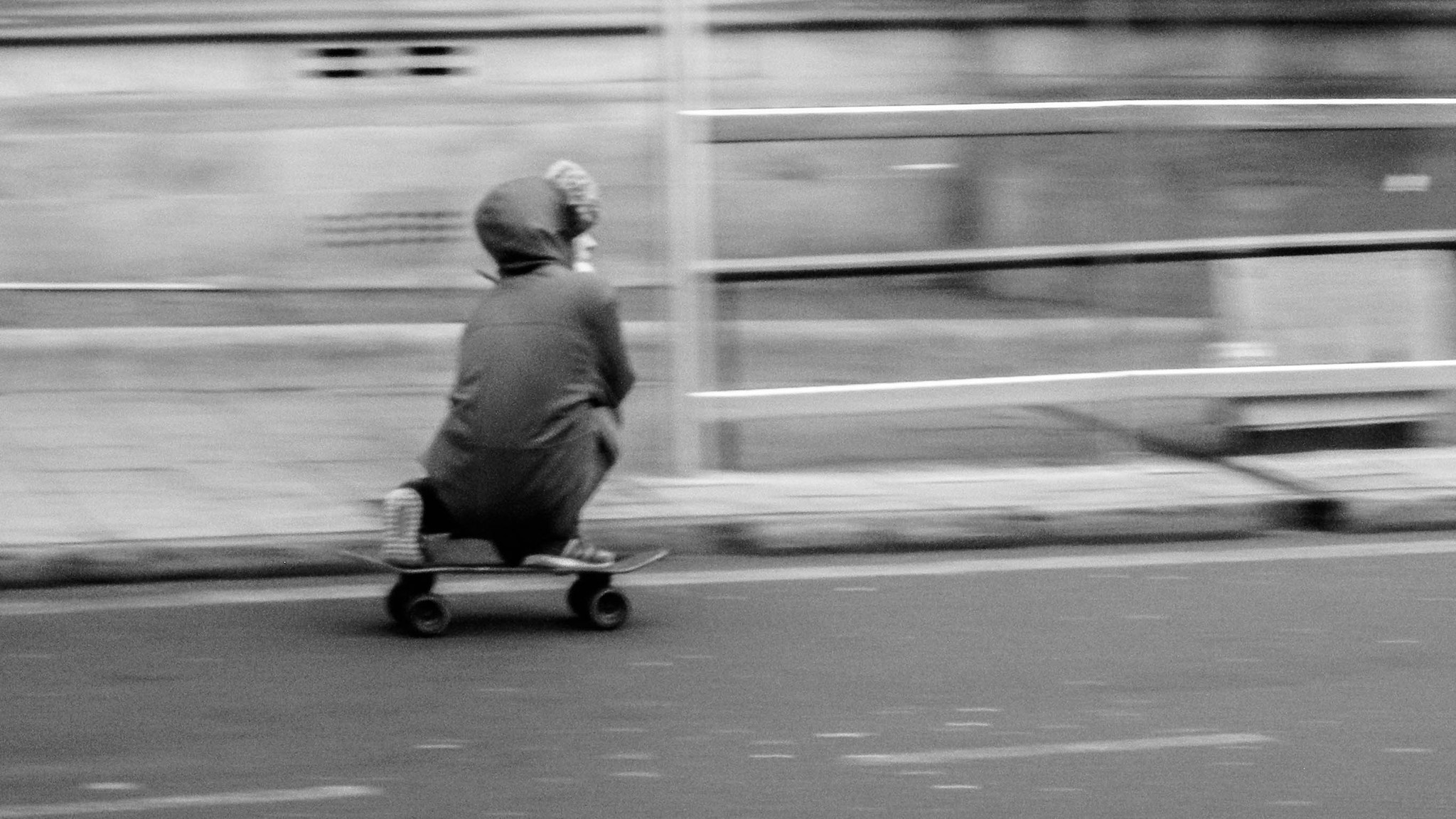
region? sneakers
[521,534,619,568]
[381,483,433,564]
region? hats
[544,161,598,237]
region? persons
[378,159,639,572]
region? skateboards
[346,548,670,636]
